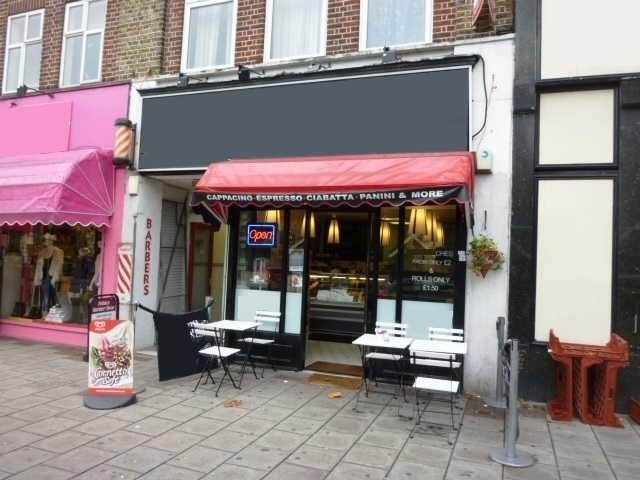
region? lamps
[231,64,265,82]
[173,74,207,89]
[16,85,55,102]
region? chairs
[188,320,242,395]
[237,310,280,380]
[426,326,464,408]
[365,322,410,398]
[407,347,463,430]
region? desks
[203,321,262,389]
[408,337,469,407]
[353,333,413,404]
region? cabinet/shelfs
[268,268,388,343]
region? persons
[20,231,36,318]
[33,233,65,320]
[85,239,103,295]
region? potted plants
[468,236,505,280]
